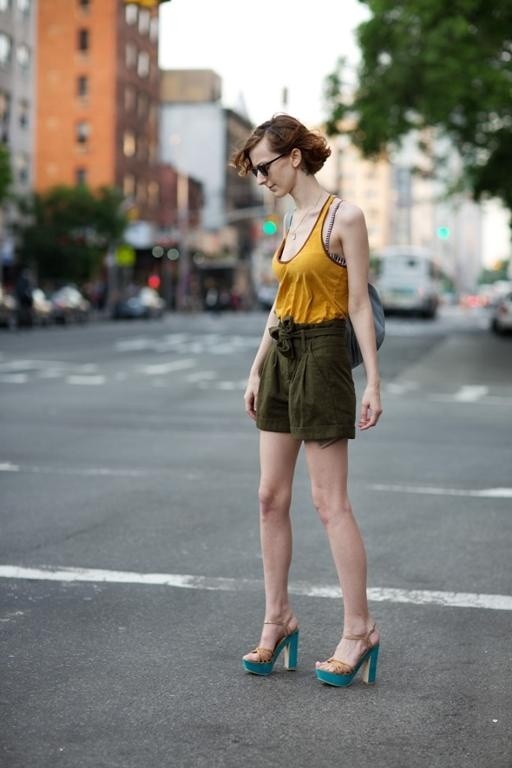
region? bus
[363,244,446,319]
[253,242,279,309]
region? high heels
[315,623,380,687]
[242,613,299,677]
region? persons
[229,115,383,688]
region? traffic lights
[262,213,282,236]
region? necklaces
[290,190,323,240]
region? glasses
[251,153,290,177]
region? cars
[0,278,170,332]
[447,279,512,337]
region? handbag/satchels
[350,282,385,369]
